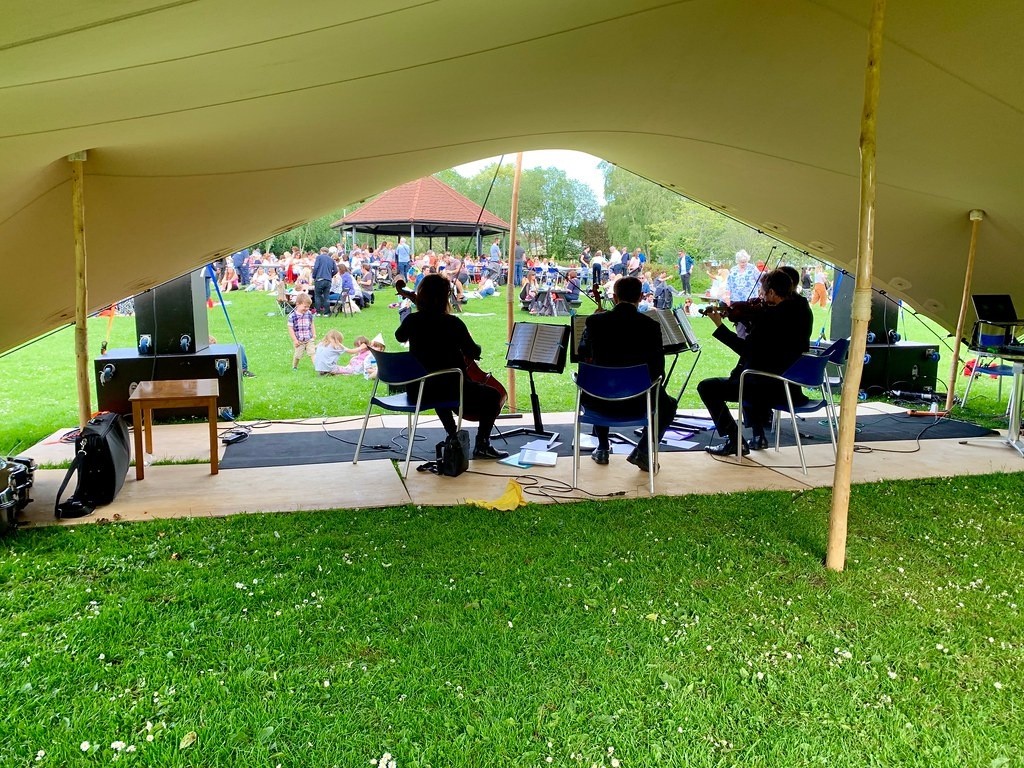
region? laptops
[971,294,1024,326]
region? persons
[106,237,830,472]
[311,247,338,316]
[287,293,316,370]
[394,273,509,460]
[674,249,694,297]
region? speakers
[809,264,939,400]
[95,266,244,422]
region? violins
[694,296,766,327]
[587,281,608,313]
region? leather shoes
[748,436,768,449]
[705,439,750,456]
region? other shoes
[626,447,661,473]
[678,291,685,296]
[324,314,328,318]
[473,439,509,460]
[243,371,257,378]
[460,295,465,299]
[474,292,483,299]
[293,367,297,370]
[590,447,611,464]
[525,295,532,301]
[314,313,321,316]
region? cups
[675,266,678,269]
[357,276,361,281]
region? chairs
[961,322,1018,416]
[352,345,463,478]
[275,282,296,316]
[572,361,662,496]
[734,338,870,477]
[440,263,562,283]
[328,286,354,318]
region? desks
[128,378,219,481]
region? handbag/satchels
[55,412,131,505]
[439,430,470,476]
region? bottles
[931,402,938,412]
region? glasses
[686,301,690,303]
[679,253,682,255]
[532,274,535,276]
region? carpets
[218,412,1001,470]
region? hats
[372,333,386,348]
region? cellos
[394,277,511,445]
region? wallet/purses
[498,260,501,264]
